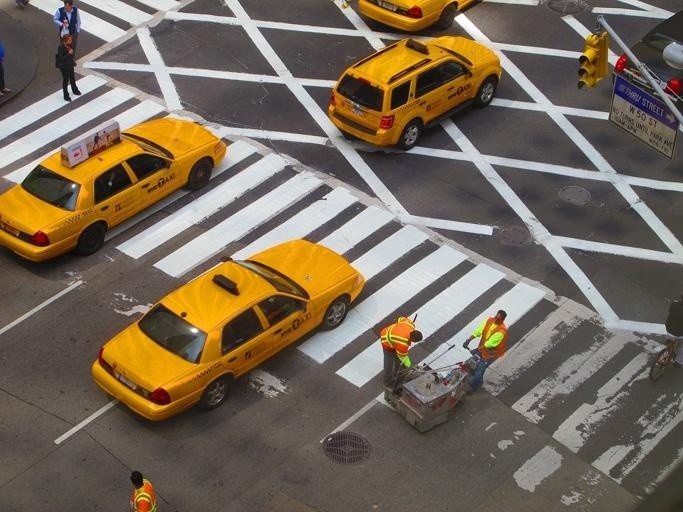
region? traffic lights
[576,30,612,94]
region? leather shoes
[63,88,81,101]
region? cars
[353,0,483,32]
[0,116,228,263]
[613,10,683,108]
[89,237,367,423]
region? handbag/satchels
[55,53,59,68]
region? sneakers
[0,87,12,97]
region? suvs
[327,32,502,150]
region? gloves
[462,339,469,348]
[469,348,479,356]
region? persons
[92,133,101,150]
[100,130,110,144]
[52,0,81,68]
[55,33,81,104]
[378,315,422,383]
[0,42,11,99]
[462,309,509,393]
[128,470,157,511]
